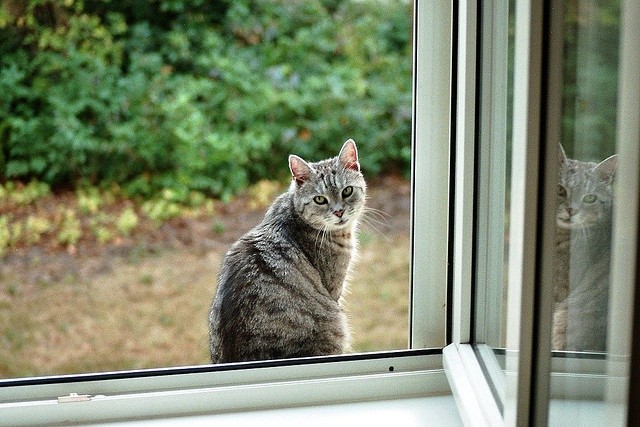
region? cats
[207,138,393,366]
[550,140,618,355]
